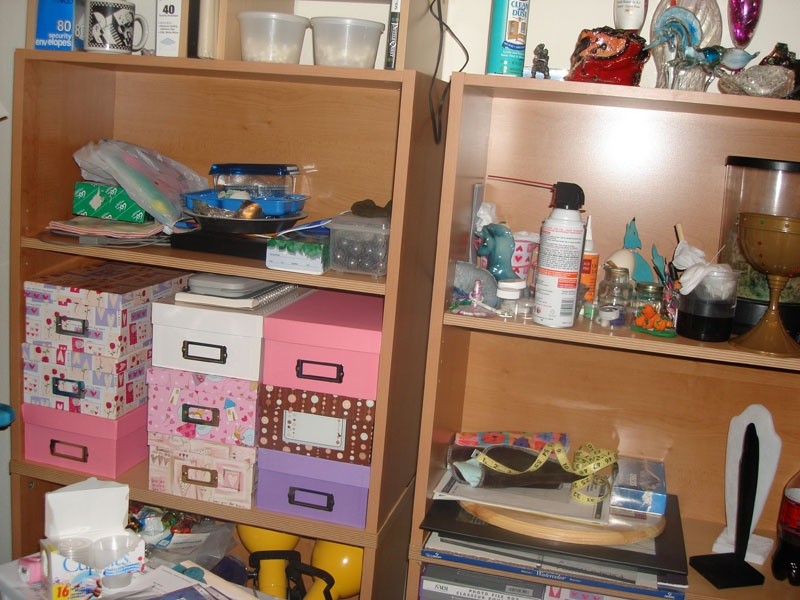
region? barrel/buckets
[716,155,800,338]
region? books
[419,461,688,600]
[97,560,257,600]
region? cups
[93,535,138,589]
[57,538,92,567]
[84,0,148,55]
[675,270,741,342]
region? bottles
[634,282,663,320]
[598,268,634,329]
[578,215,599,315]
[771,470,800,586]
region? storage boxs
[20,211,391,531]
[611,456,666,516]
[35,0,87,51]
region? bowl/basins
[238,11,309,64]
[310,17,385,70]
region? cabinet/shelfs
[407,72,800,600]
[12,0,450,600]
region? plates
[180,188,311,215]
[184,208,307,234]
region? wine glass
[729,213,800,358]
